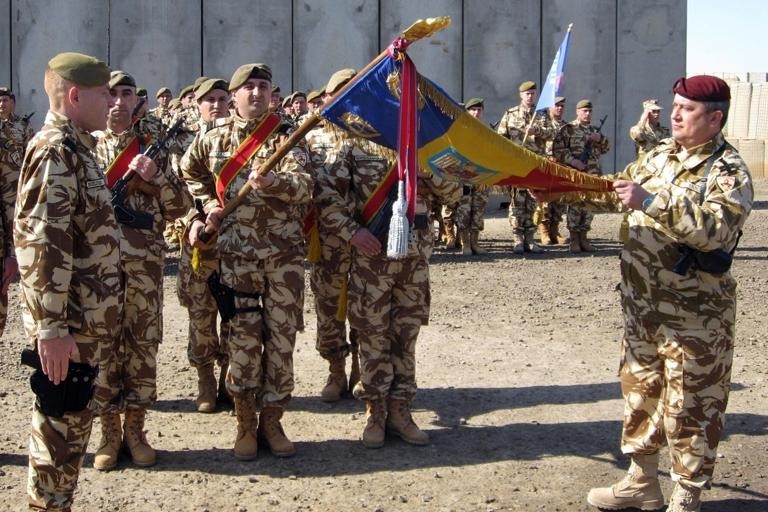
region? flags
[316,51,623,214]
[536,32,570,112]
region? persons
[310,69,366,402]
[526,76,753,511]
[0,192,18,343]
[12,52,121,511]
[173,78,235,413]
[314,138,467,449]
[89,70,195,467]
[180,62,316,459]
[630,98,669,158]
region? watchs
[642,192,655,211]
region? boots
[347,347,366,397]
[91,408,125,471]
[664,477,703,511]
[586,451,665,510]
[261,397,297,456]
[218,362,240,403]
[194,362,219,414]
[361,399,388,448]
[122,405,161,467]
[318,358,348,401]
[391,395,433,446]
[230,388,263,461]
[434,215,604,260]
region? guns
[109,114,186,231]
[581,113,608,166]
[207,268,234,322]
[21,351,61,415]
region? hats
[169,77,230,110]
[136,88,147,98]
[1,87,16,103]
[271,86,325,106]
[465,95,484,108]
[228,63,273,89]
[326,67,357,93]
[156,88,171,99]
[671,71,731,103]
[519,80,595,111]
[641,97,664,111]
[49,53,112,87]
[106,70,137,88]
